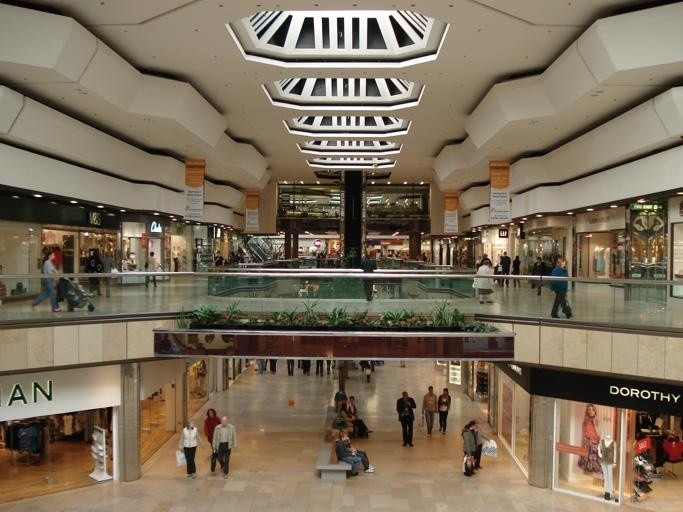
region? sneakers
[426,430,447,439]
[462,464,482,477]
[364,464,376,472]
[350,470,359,477]
[185,467,232,480]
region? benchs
[316,405,354,480]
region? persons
[438,387,451,434]
[212,416,237,479]
[578,404,603,480]
[336,396,375,475]
[310,248,572,319]
[178,420,206,478]
[256,359,384,383]
[32,248,244,312]
[421,386,438,439]
[396,391,417,447]
[597,435,617,499]
[462,420,490,476]
[204,408,221,446]
[592,246,610,278]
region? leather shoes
[479,300,484,304]
[486,301,494,303]
[408,442,413,447]
[402,443,407,447]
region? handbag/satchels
[175,448,188,468]
[462,454,475,473]
[482,439,498,458]
[417,414,424,428]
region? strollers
[56,276,94,312]
